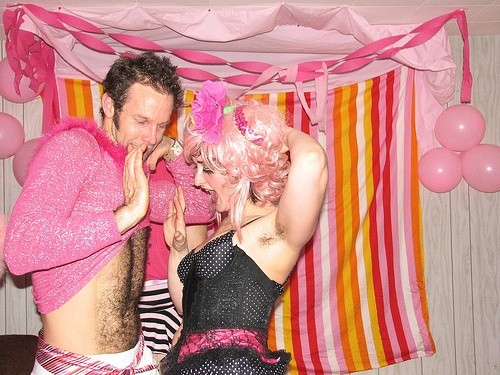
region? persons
[158,102,328,375]
[2,50,217,375]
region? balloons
[417,147,463,193]
[0,54,48,104]
[434,104,487,152]
[0,111,26,159]
[459,144,500,193]
[13,136,47,187]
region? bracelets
[164,138,184,163]
